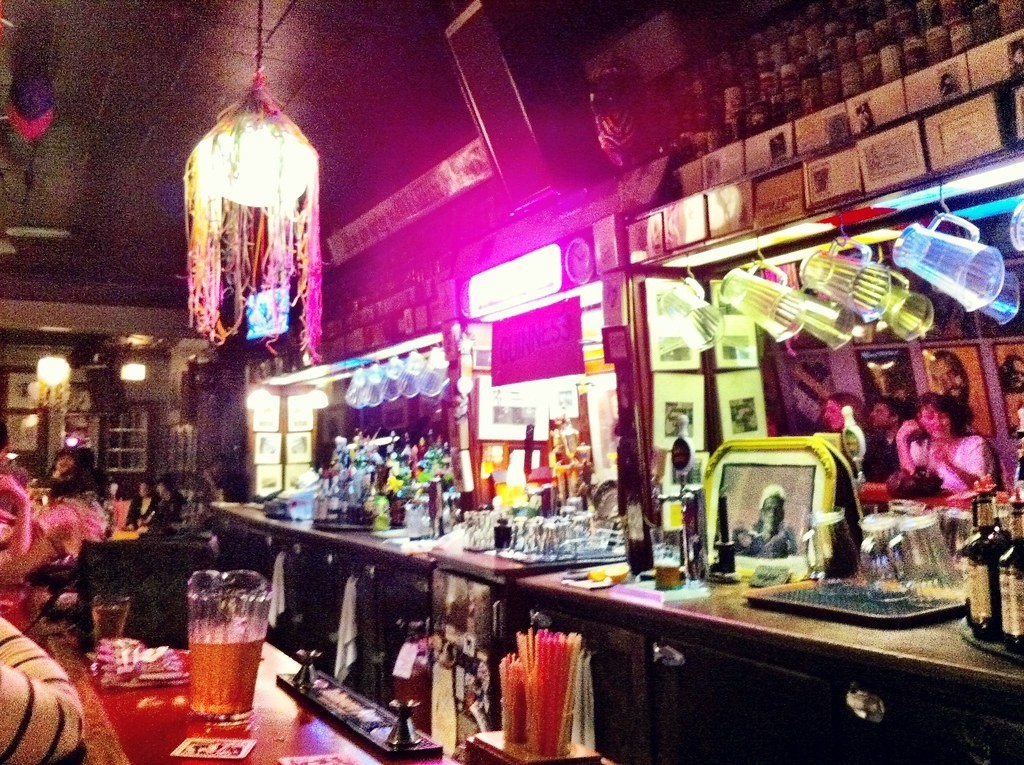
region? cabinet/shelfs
[208,501,507,729]
[513,563,1024,765]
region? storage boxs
[465,729,602,765]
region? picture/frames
[855,347,920,421]
[759,356,789,437]
[781,291,830,350]
[916,274,979,341]
[981,263,1024,339]
[784,351,838,434]
[852,312,908,347]
[857,118,927,194]
[922,92,1007,172]
[920,343,998,440]
[704,436,837,582]
[991,339,1024,440]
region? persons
[749,482,797,559]
[930,350,988,437]
[0,421,187,563]
[0,609,84,765]
[823,392,998,494]
[669,435,700,484]
[998,353,1024,434]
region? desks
[83,641,463,765]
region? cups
[651,197,1024,348]
[797,499,968,609]
[344,348,449,411]
[650,526,683,592]
[184,568,273,727]
[90,594,130,648]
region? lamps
[181,0,325,368]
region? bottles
[690,1,1024,161]
[959,489,1024,656]
[310,427,453,534]
[461,506,594,564]
